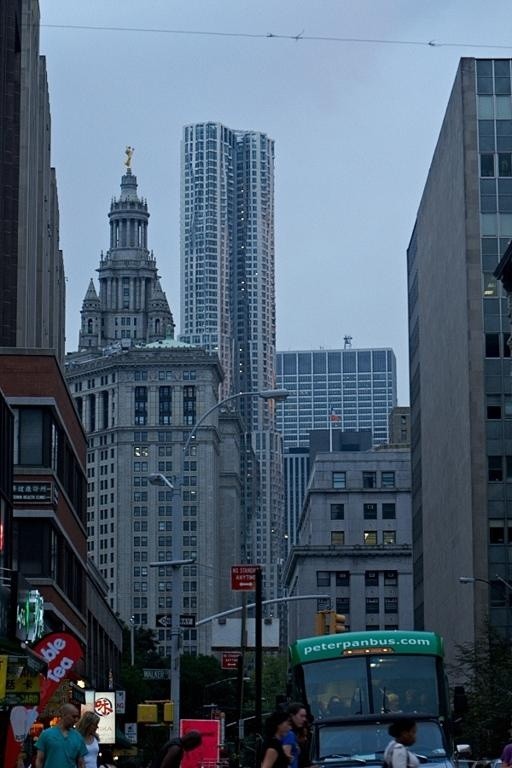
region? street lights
[146,388,289,739]
[201,676,251,719]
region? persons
[256,711,290,768]
[150,731,201,768]
[280,702,307,768]
[75,710,100,768]
[34,702,89,768]
[381,718,420,768]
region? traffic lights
[336,614,347,632]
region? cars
[299,713,473,768]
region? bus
[285,629,466,721]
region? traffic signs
[222,651,243,671]
[230,564,262,593]
